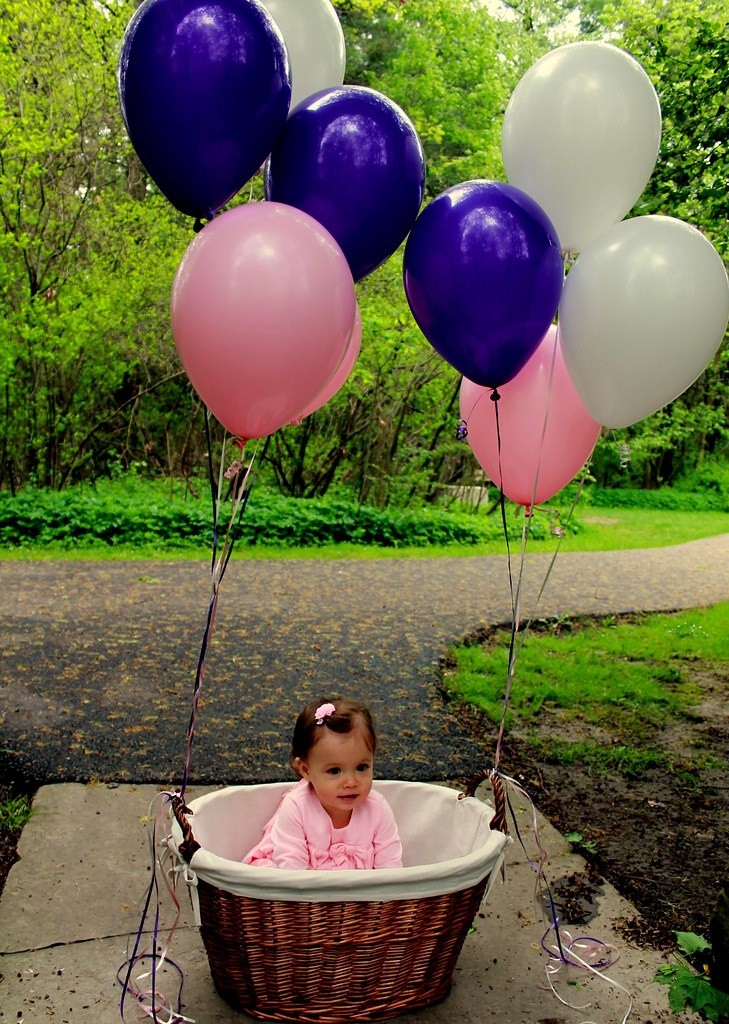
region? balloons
[560,213,729,431]
[458,324,604,507]
[262,85,426,285]
[500,42,661,252]
[258,0,346,116]
[118,0,292,193]
[404,178,565,391]
[167,200,362,450]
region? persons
[239,696,404,874]
